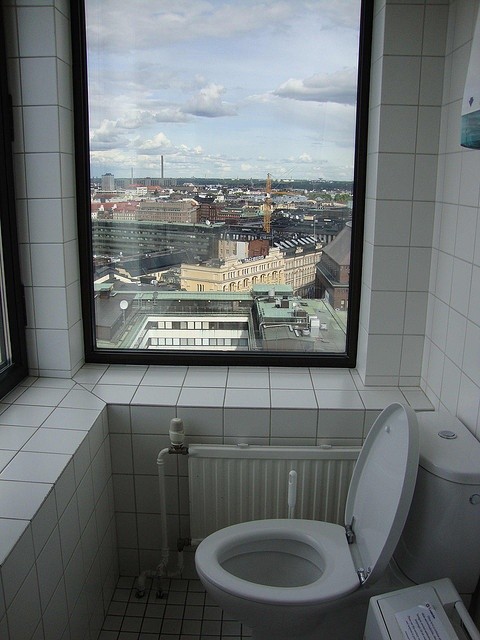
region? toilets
[195,404,480,630]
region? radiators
[187,444,364,547]
